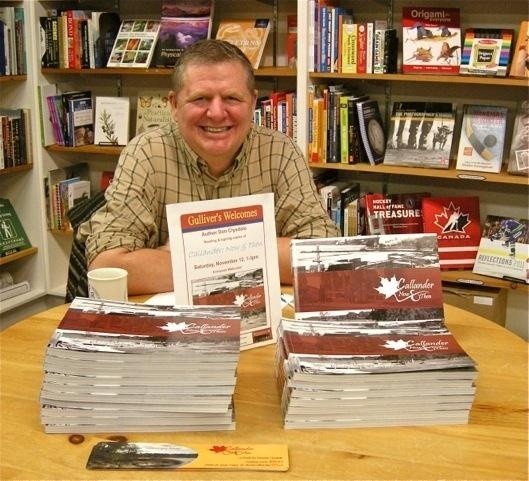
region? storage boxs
[442,286,507,328]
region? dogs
[432,126,454,151]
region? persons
[84,41,342,297]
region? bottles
[473,39,500,68]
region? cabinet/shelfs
[0,0,528,314]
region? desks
[0,285,529,481]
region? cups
[86,267,129,304]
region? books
[0,5,33,297]
[37,83,175,148]
[308,0,528,286]
[43,162,115,231]
[39,0,297,71]
[273,233,481,431]
[37,299,241,434]
[252,90,298,147]
